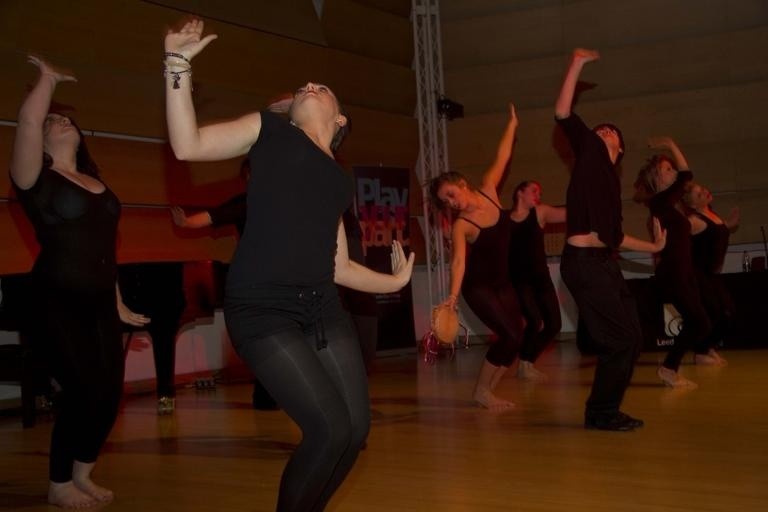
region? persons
[169,154,283,412]
[635,138,741,387]
[9,53,155,509]
[504,181,567,379]
[163,18,418,510]
[554,49,667,431]
[429,104,522,407]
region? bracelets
[162,50,196,93]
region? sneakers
[158,397,175,414]
[586,411,643,432]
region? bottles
[742,249,750,273]
